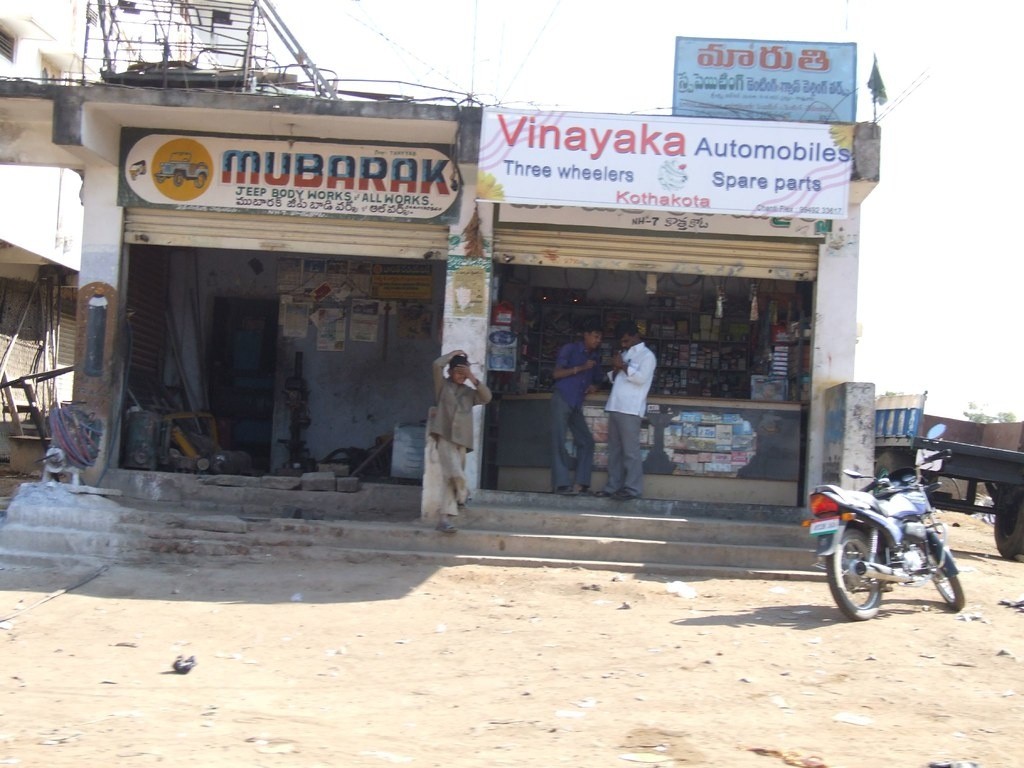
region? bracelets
[574,366,576,373]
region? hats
[450,355,467,365]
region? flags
[867,61,887,104]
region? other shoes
[594,490,609,496]
[613,489,632,499]
[436,520,456,535]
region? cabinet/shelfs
[485,303,810,480]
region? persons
[550,322,608,494]
[433,350,492,532]
[596,320,656,500]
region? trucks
[874,389,1024,563]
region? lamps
[645,273,657,295]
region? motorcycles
[801,424,967,620]
